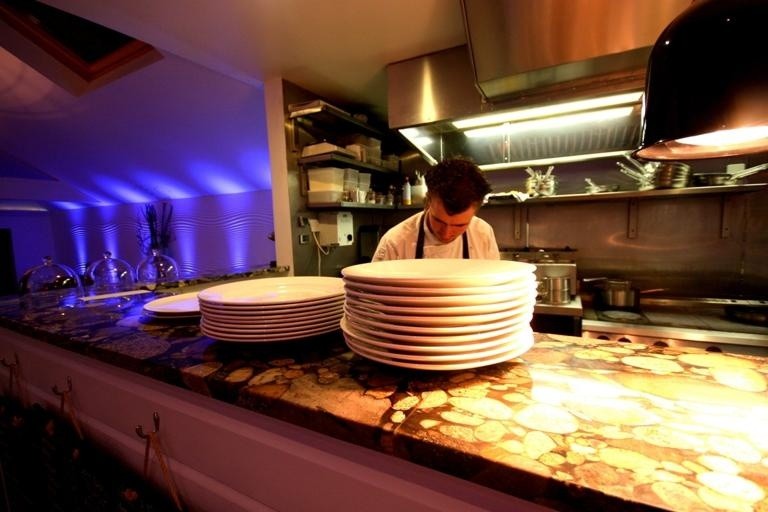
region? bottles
[385,176,412,206]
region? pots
[584,176,619,192]
[523,165,559,196]
[535,277,571,305]
[603,285,667,309]
[618,151,690,190]
[692,162,767,185]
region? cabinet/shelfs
[288,105,403,212]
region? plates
[340,257,539,373]
[142,290,200,320]
[197,276,344,343]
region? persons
[371,158,500,263]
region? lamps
[630,0,767,163]
